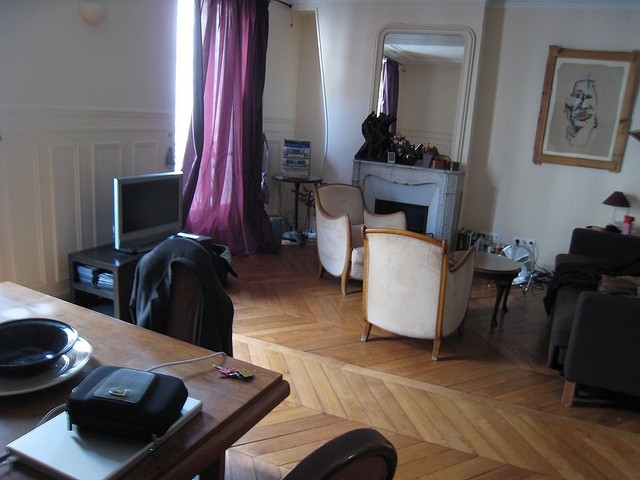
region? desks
[69,231,212,321]
[273,174,323,248]
[447,251,521,326]
[0,281,290,479]
[569,227,640,260]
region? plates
[0,336,93,396]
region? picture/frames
[532,45,640,173]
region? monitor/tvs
[111,171,184,254]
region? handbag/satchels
[67,365,188,441]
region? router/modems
[5,397,202,480]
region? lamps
[602,191,630,232]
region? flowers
[387,132,410,158]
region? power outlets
[512,237,536,248]
[479,232,501,243]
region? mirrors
[369,24,477,171]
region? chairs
[281,427,397,479]
[361,225,475,361]
[128,238,234,358]
[312,183,406,296]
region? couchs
[544,254,639,409]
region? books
[77,266,114,289]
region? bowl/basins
[0,318,78,379]
